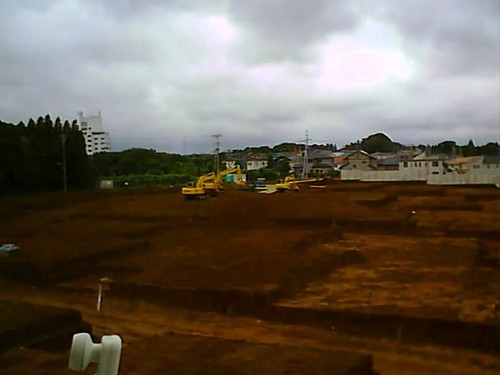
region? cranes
[295,130,332,179]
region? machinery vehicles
[203,164,244,193]
[275,175,300,193]
[182,172,219,200]
